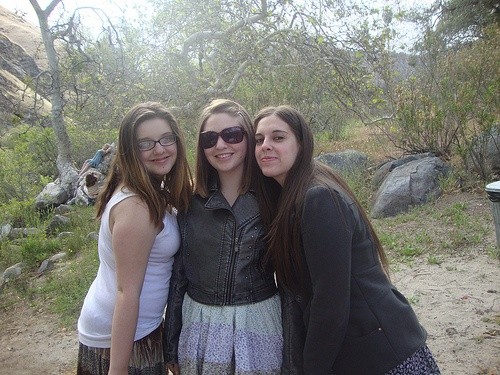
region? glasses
[199,127,249,149]
[136,134,176,151]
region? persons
[252,105,440,375]
[163,98,284,375]
[77,101,194,375]
[78,140,116,179]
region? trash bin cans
[485,180,500,257]
